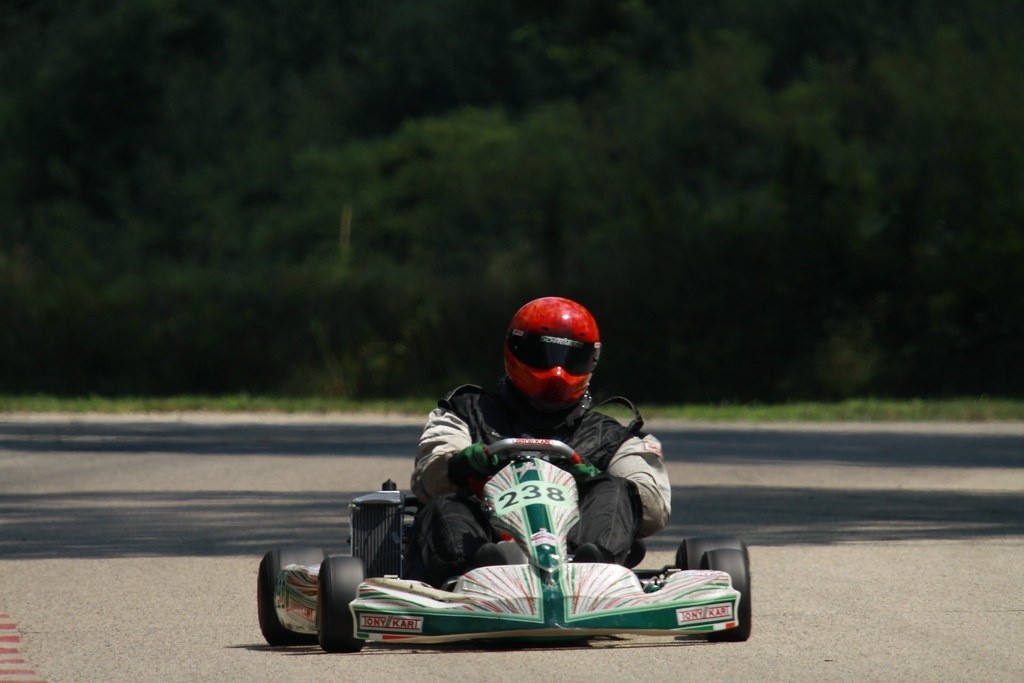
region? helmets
[503,297,601,409]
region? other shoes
[573,542,605,563]
[473,542,507,569]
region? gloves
[561,456,601,484]
[448,442,498,483]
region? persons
[404,295,674,595]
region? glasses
[509,328,600,375]
